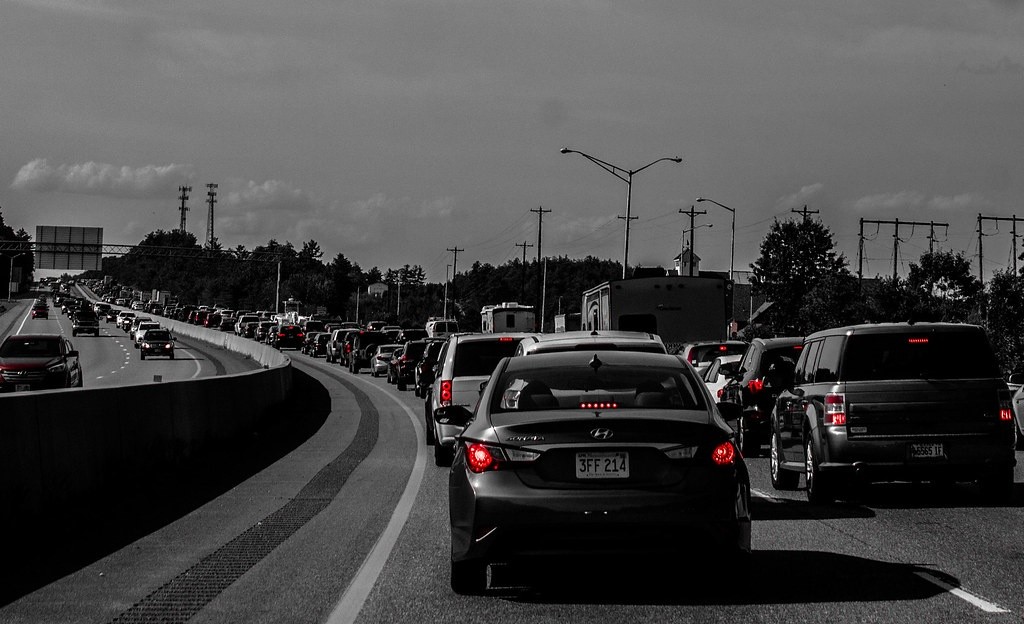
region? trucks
[51,283,59,292]
[103,276,112,284]
[140,289,170,304]
[480,301,536,334]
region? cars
[1009,372,1024,452]
[447,352,752,595]
[363,321,428,344]
[106,310,121,322]
[94,302,111,318]
[244,320,305,351]
[86,280,133,306]
[32,301,49,319]
[388,347,402,384]
[68,305,92,319]
[0,335,83,393]
[131,300,235,333]
[700,354,745,406]
[39,277,91,307]
[302,320,359,358]
[115,311,135,328]
[369,344,404,377]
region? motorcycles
[123,319,132,333]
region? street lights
[560,147,682,280]
[680,223,713,276]
[0,252,26,302]
[696,196,736,340]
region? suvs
[130,316,153,340]
[424,333,533,466]
[141,328,177,361]
[398,340,436,391]
[233,315,259,337]
[134,321,160,349]
[415,341,446,398]
[325,328,360,364]
[720,336,811,456]
[771,321,1017,509]
[673,341,751,368]
[340,331,388,374]
[71,308,99,337]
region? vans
[426,319,459,342]
[514,329,669,359]
